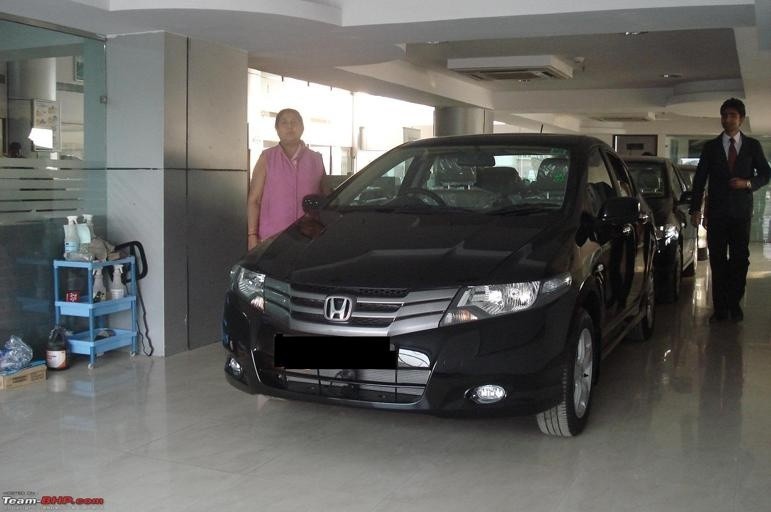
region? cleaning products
[109,264,127,300]
[64,216,80,260]
[92,266,107,303]
[82,213,96,242]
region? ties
[728,138,738,172]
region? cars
[677,156,709,262]
[214,132,664,441]
[614,150,699,302]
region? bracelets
[248,233,258,236]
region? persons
[247,107,330,252]
[689,98,770,323]
[9,142,22,158]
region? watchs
[746,180,751,189]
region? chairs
[419,154,570,209]
[637,170,662,196]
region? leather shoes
[709,310,728,321]
[729,303,743,321]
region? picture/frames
[31,98,62,151]
